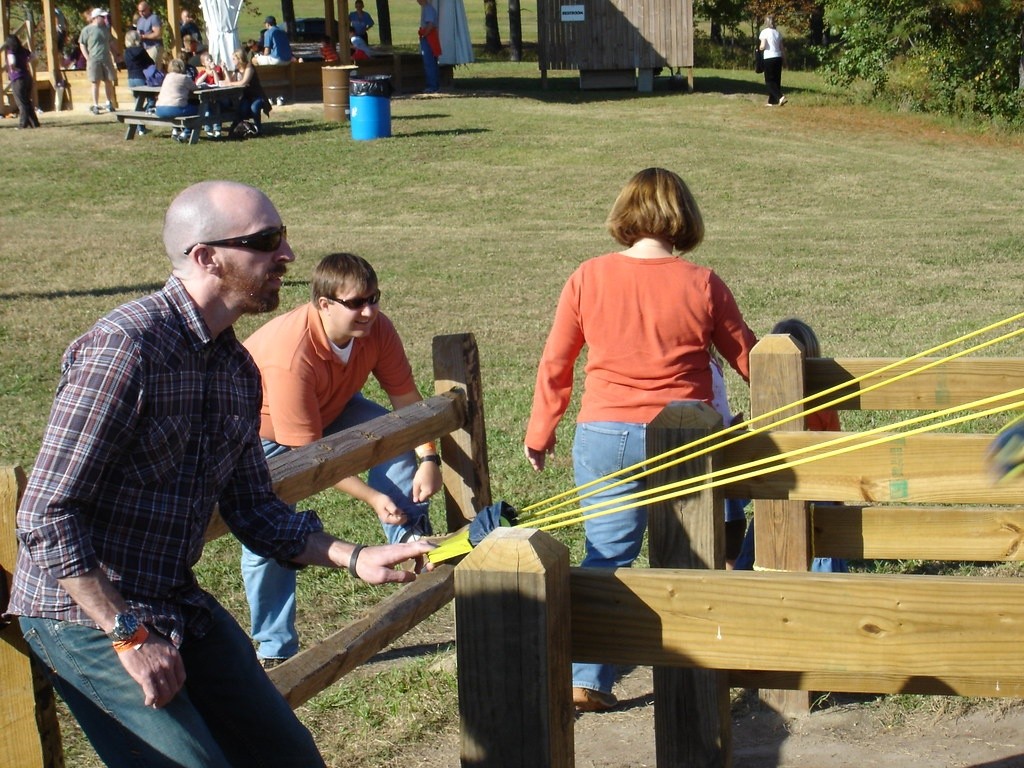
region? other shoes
[206,130,221,140]
[139,128,152,136]
[171,129,189,144]
[779,95,788,106]
[90,105,99,115]
[106,100,114,111]
[268,95,285,106]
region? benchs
[115,111,201,129]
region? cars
[278,16,338,58]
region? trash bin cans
[321,64,358,122]
[349,75,393,140]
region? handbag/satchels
[755,46,764,73]
[143,64,164,87]
[233,120,260,139]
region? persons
[180,9,202,64]
[416,0,441,93]
[78,8,119,114]
[241,252,442,669]
[321,0,374,66]
[156,51,272,143]
[125,1,162,135]
[524,168,759,709]
[5,35,40,128]
[1,179,437,768]
[245,16,292,66]
[759,15,786,107]
[710,319,849,572]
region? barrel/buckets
[321,65,360,123]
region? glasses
[183,225,286,255]
[319,289,381,309]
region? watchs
[420,454,441,466]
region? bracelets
[112,623,149,653]
[350,545,368,580]
[106,607,140,642]
[107,23,112,28]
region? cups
[219,81,226,85]
[201,83,206,88]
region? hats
[264,16,276,26]
[91,8,109,17]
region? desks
[125,84,264,146]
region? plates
[207,85,219,88]
[219,85,231,88]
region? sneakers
[573,686,617,711]
